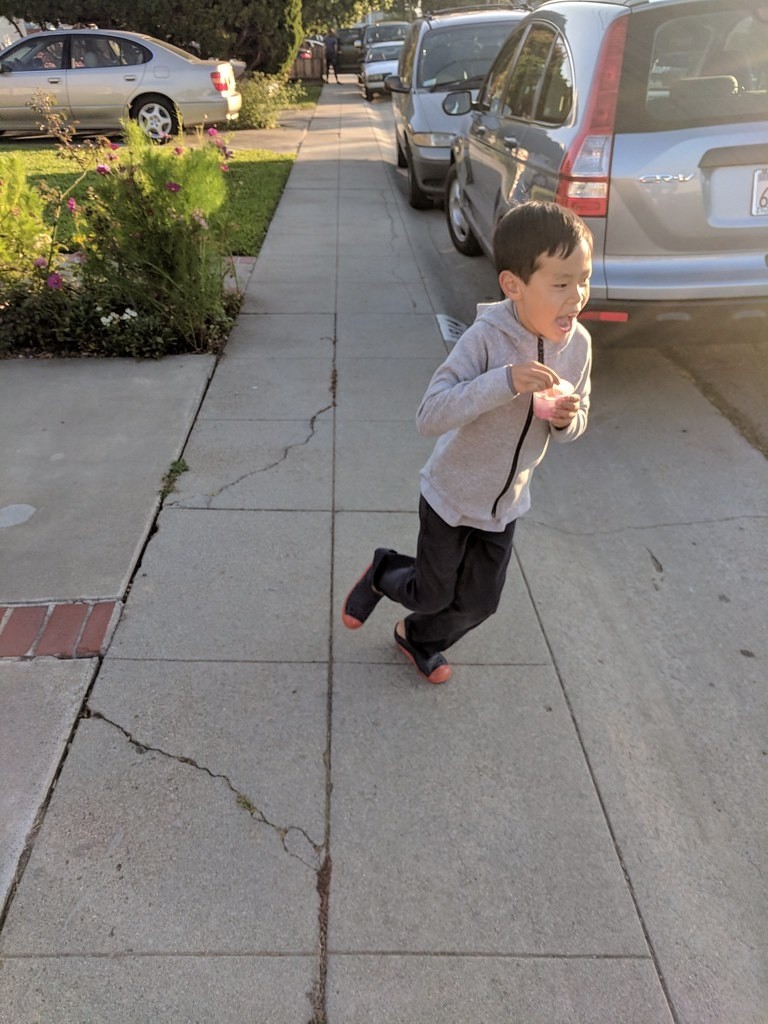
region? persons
[341,200,593,683]
[307,29,324,42]
[322,28,343,85]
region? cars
[333,21,411,55]
[0,26,242,145]
[443,0,768,347]
[359,40,405,102]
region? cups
[533,379,575,420]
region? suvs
[384,2,535,210]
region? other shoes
[342,564,383,629]
[394,622,450,684]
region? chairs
[372,52,385,62]
[669,50,752,97]
[83,52,104,66]
[427,44,456,70]
